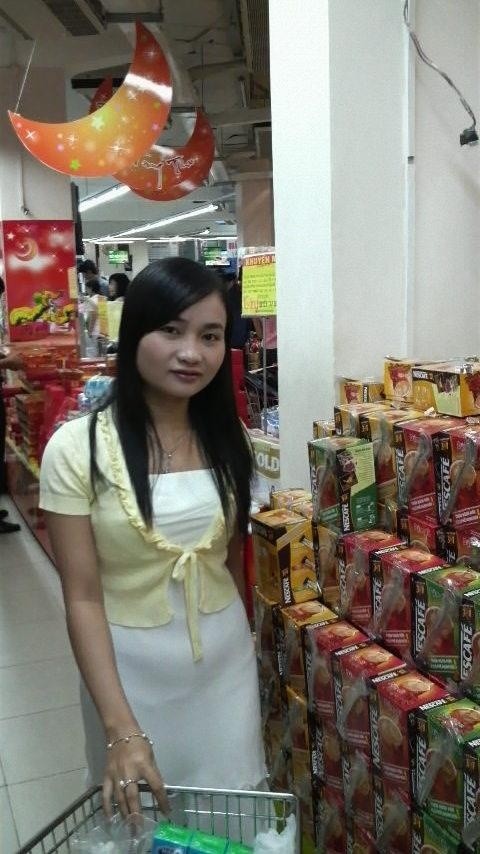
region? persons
[0,278,29,535]
[55,254,279,428]
[32,253,278,847]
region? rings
[118,776,136,791]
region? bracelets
[102,732,154,752]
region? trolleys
[244,364,277,428]
[17,783,300,854]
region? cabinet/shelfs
[0,436,76,578]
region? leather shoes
[0,521,21,533]
